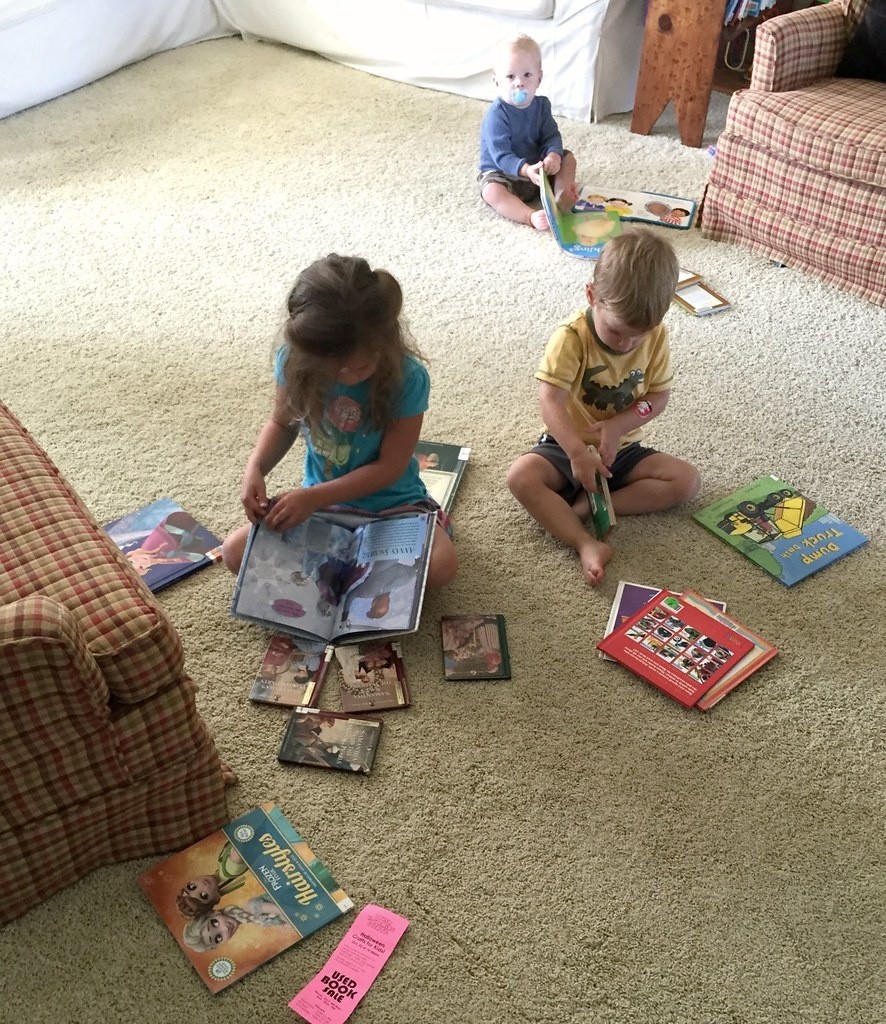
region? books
[443,613,512,680]
[100,495,223,596]
[232,507,441,641]
[276,706,383,775]
[595,471,867,709]
[331,643,409,713]
[534,162,731,315]
[136,799,355,996]
[247,637,329,707]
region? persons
[287,639,327,684]
[508,227,700,584]
[222,250,460,601]
[476,32,579,230]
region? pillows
[834,0,886,83]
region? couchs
[703,0,886,306]
[0,400,235,924]
